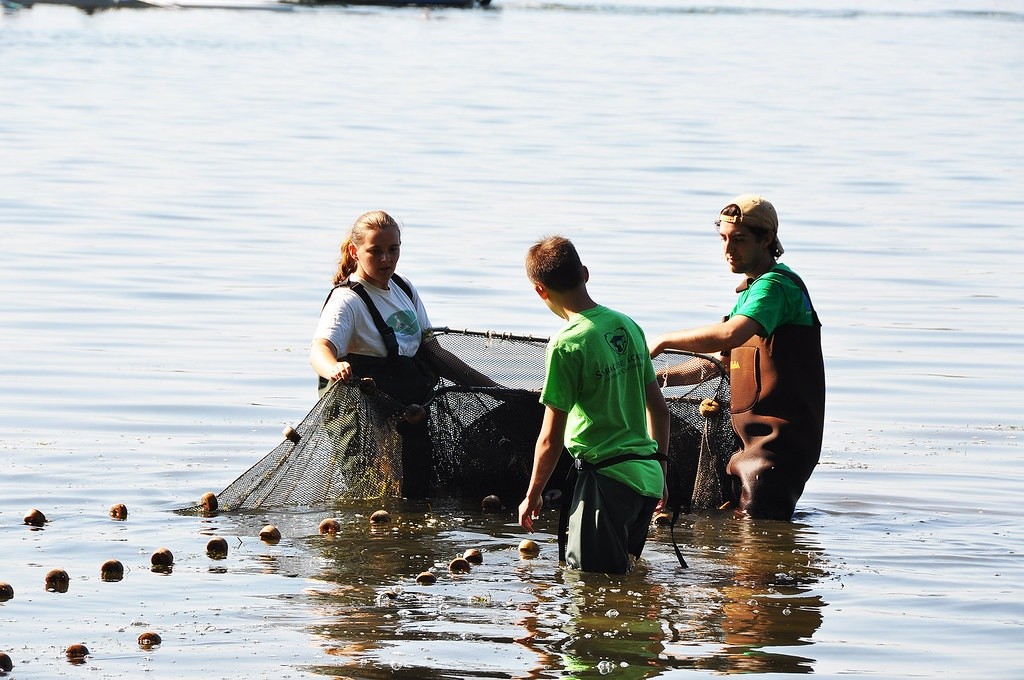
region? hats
[720,198,785,254]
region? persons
[519,234,669,573]
[309,210,514,498]
[647,199,825,518]
